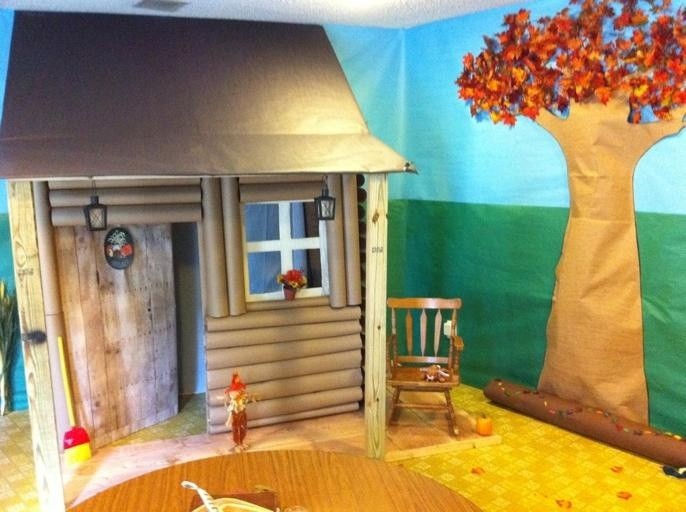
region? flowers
[277,270,307,292]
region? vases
[282,287,295,301]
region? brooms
[55,337,93,465]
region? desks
[65,449,482,512]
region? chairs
[385,297,464,438]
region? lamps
[314,175,336,221]
[83,180,108,232]
